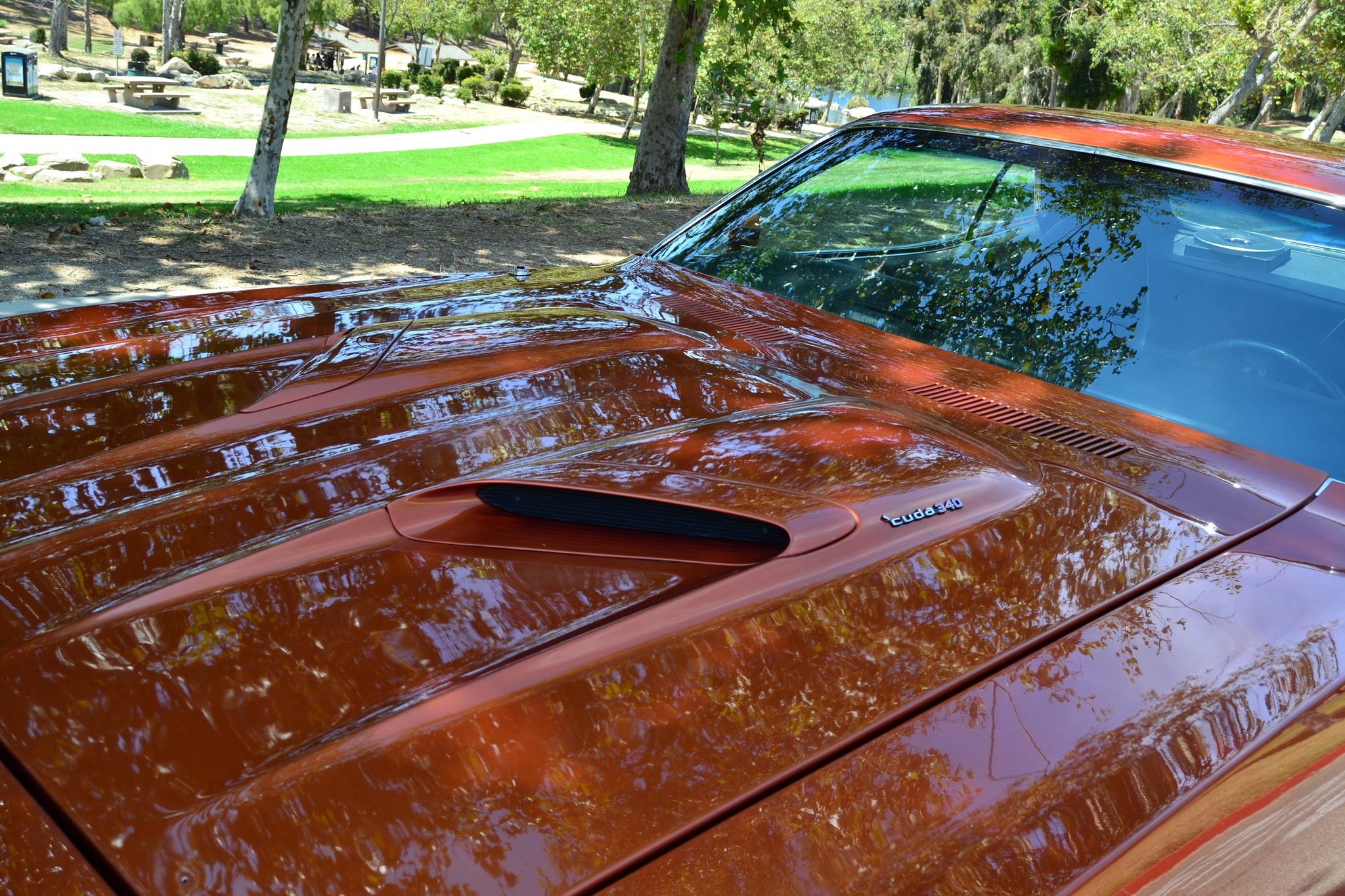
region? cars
[0,102,1344,896]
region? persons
[306,52,335,71]
[381,65,386,72]
[341,53,344,69]
[372,64,377,75]
[350,64,360,70]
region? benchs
[205,36,215,39]
[0,36,18,40]
[268,45,276,49]
[1032,234,1345,405]
[354,94,388,99]
[383,99,417,105]
[219,38,232,42]
[95,85,154,90]
[132,92,190,98]
[306,62,322,72]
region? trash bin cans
[139,35,154,46]
[1,49,38,98]
[216,43,223,54]
[362,53,368,60]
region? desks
[208,32,229,45]
[0,29,13,45]
[107,76,181,110]
[359,88,411,113]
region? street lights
[897,22,915,108]
